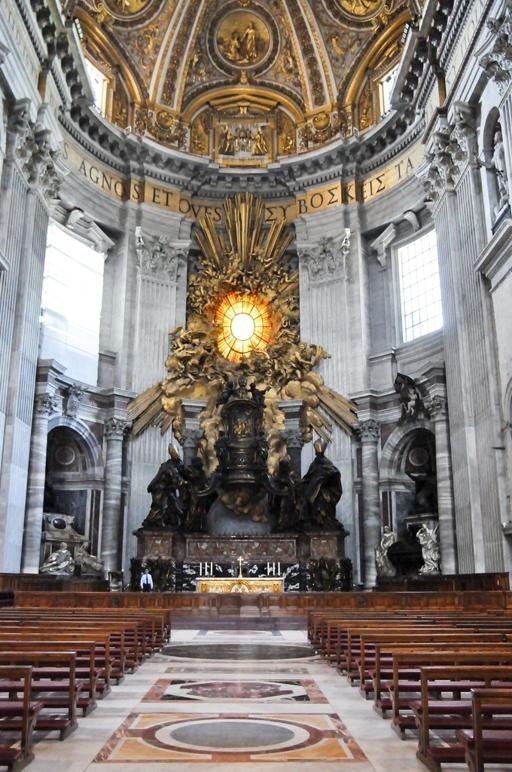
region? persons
[115,13,351,158]
[45,541,74,576]
[416,522,440,572]
[140,567,153,592]
[376,526,396,577]
[167,253,320,394]
[138,434,344,532]
[406,387,418,414]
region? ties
[146,576,148,586]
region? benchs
[1,606,173,772]
[304,608,511,771]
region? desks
[193,576,287,596]
